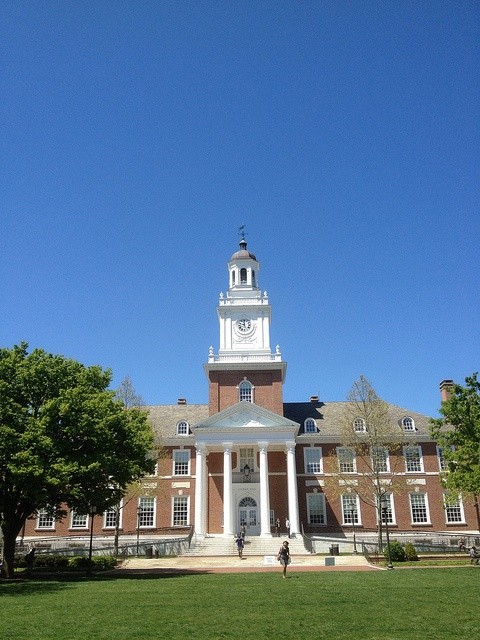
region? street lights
[84,500,97,577]
[348,500,358,556]
[379,494,394,571]
[134,505,144,557]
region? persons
[241,525,246,538]
[285,518,290,538]
[236,536,244,559]
[276,518,281,537]
[26,547,36,570]
[277,542,290,578]
[471,547,478,564]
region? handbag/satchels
[277,554,281,561]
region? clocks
[236,317,254,334]
